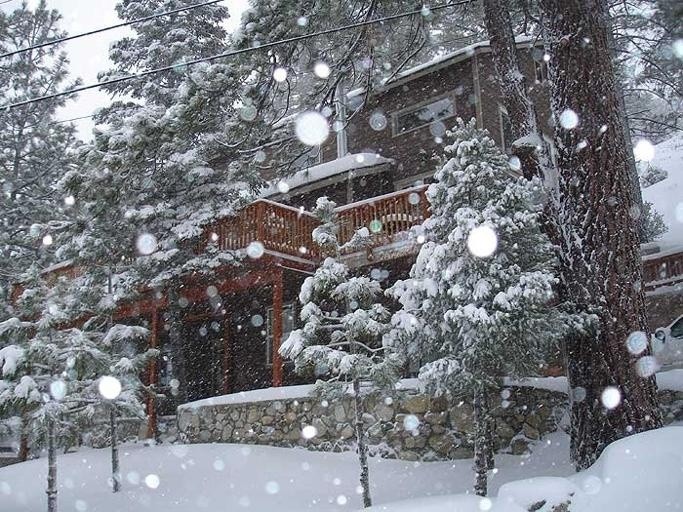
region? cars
[651,314,683,356]
